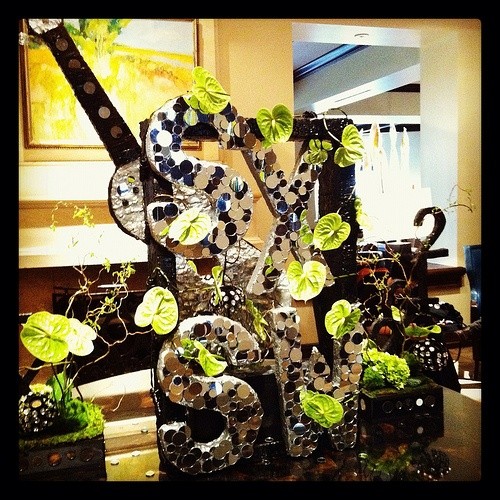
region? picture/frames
[21,18,204,154]
